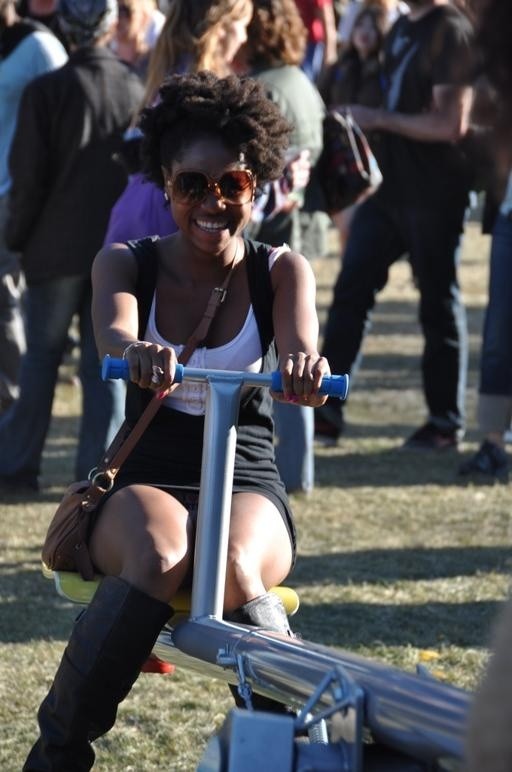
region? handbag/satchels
[300,107,384,214]
[42,481,100,582]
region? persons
[2,1,511,492]
[21,68,339,772]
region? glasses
[167,168,257,205]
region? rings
[152,366,167,385]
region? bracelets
[122,342,143,360]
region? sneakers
[455,440,511,475]
[401,421,460,452]
[312,410,343,447]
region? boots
[22,575,175,772]
[223,593,315,738]
